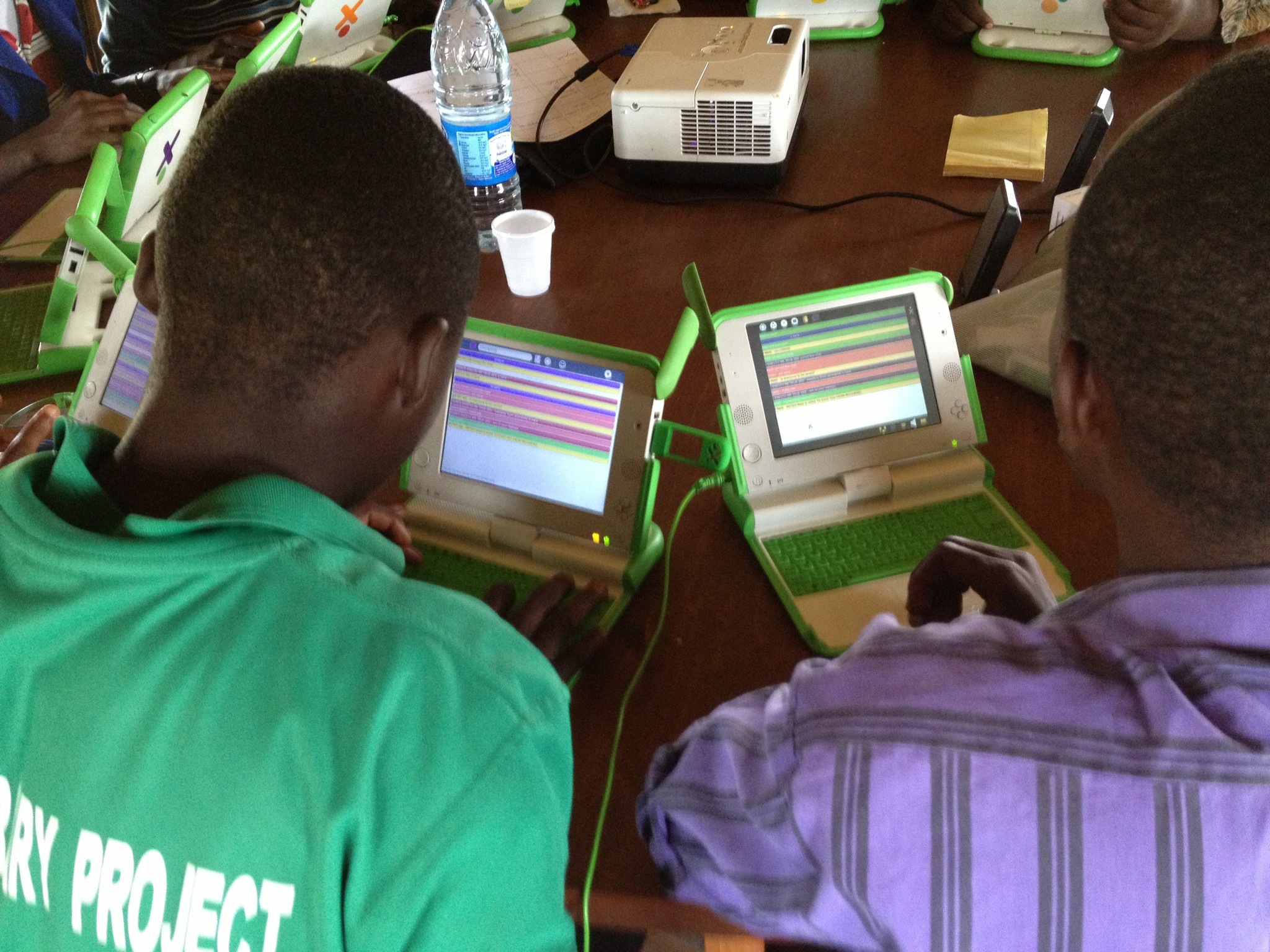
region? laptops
[681,262,1076,658]
[746,0,905,40]
[971,0,1122,67]
[398,306,731,690]
[0,0,581,431]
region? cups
[491,209,556,297]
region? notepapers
[943,107,1049,182]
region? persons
[0,404,62,469]
[0,0,303,178]
[636,48,1270,952]
[931,0,1270,51]
[0,61,586,952]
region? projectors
[611,17,810,185]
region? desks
[0,0,1270,952]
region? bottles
[430,1,521,253]
